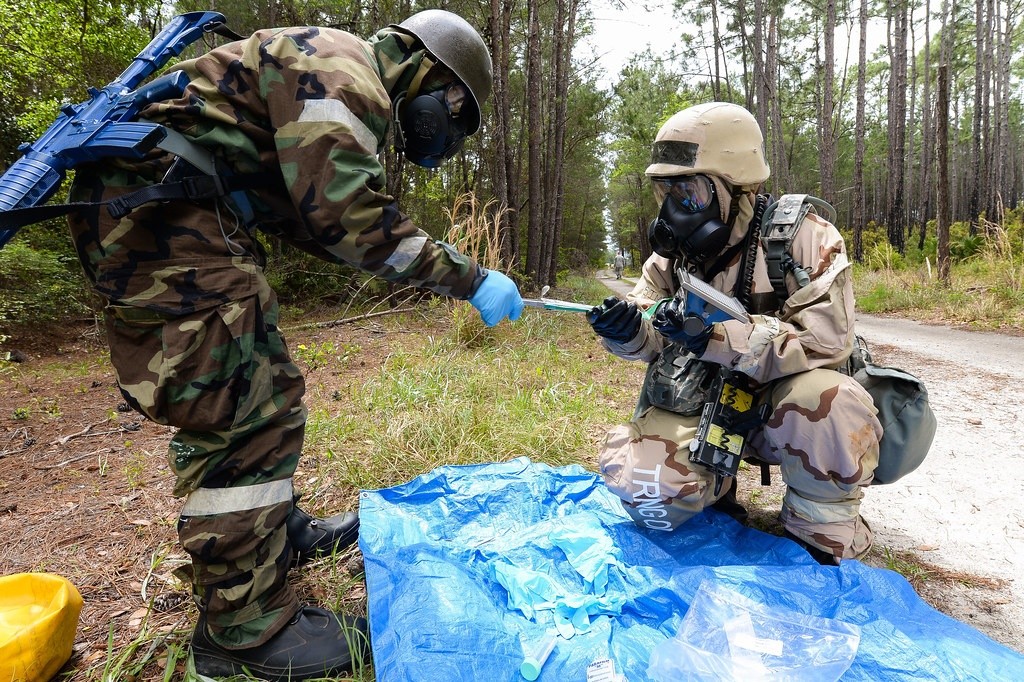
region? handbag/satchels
[846,335,937,485]
[647,341,720,417]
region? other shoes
[713,475,748,523]
[780,526,837,566]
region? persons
[589,102,885,565]
[67,8,525,678]
[614,252,625,279]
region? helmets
[645,102,770,185]
[388,9,494,135]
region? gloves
[468,270,524,327]
[586,295,642,344]
[653,296,715,358]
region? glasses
[445,81,472,118]
[652,176,716,212]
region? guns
[0,10,227,247]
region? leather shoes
[191,604,373,682]
[287,505,359,566]
